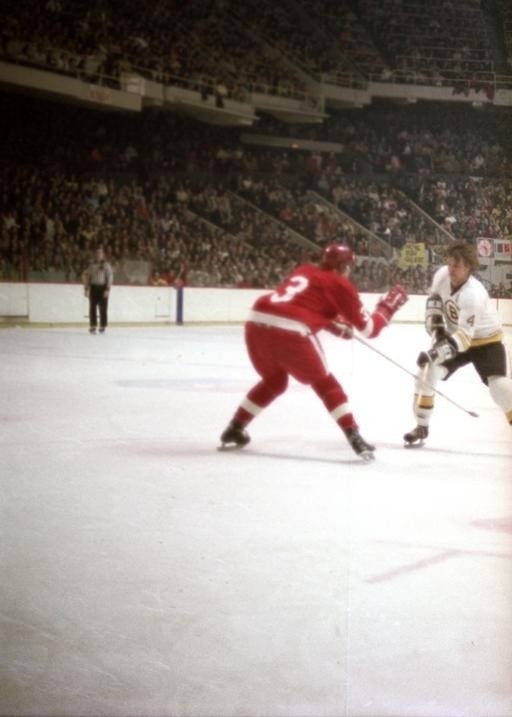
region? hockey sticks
[349,329,480,418]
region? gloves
[329,316,353,339]
[430,326,444,343]
[417,346,443,370]
[377,284,407,315]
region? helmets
[323,244,357,267]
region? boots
[221,419,249,445]
[345,427,375,456]
[402,425,427,443]
[89,326,106,331]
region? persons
[84,247,113,334]
[219,244,408,456]
[0,1,512,298]
[403,240,512,443]
